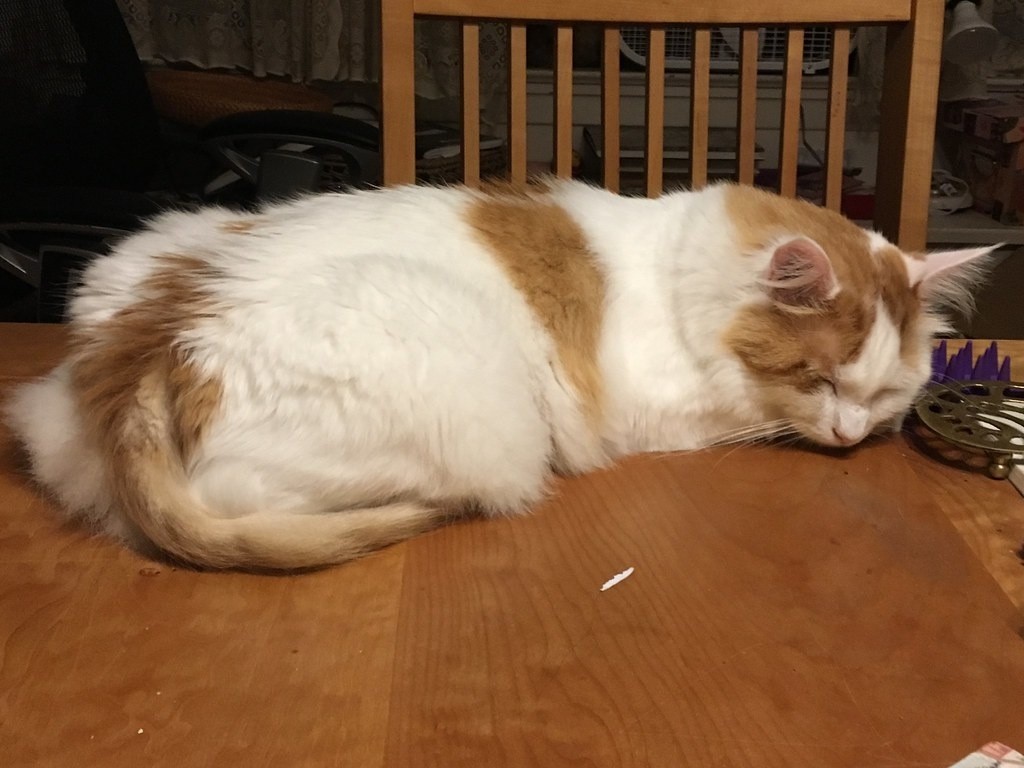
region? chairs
[381,0,945,254]
[0,0,386,323]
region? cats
[7,175,1010,571]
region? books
[360,120,504,160]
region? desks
[0,321,1024,768]
